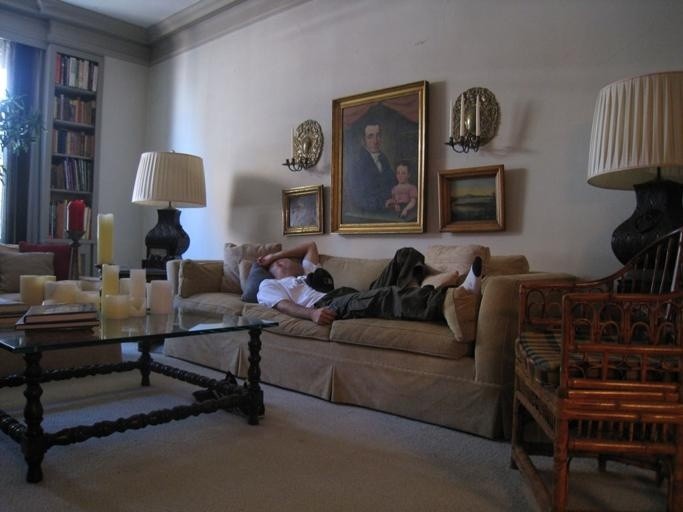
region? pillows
[18,241,70,283]
[1,253,56,294]
[217,240,285,306]
[421,242,490,286]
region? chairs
[1,240,122,383]
[505,229,682,511]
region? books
[57,244,93,279]
[50,196,91,240]
[52,159,93,194]
[14,315,101,330]
[49,126,94,159]
[51,92,97,124]
[23,301,99,323]
[53,51,98,92]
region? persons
[252,237,482,327]
[342,116,416,218]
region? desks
[107,264,172,354]
[599,273,681,452]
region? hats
[304,268,334,292]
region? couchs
[166,254,579,440]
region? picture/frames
[437,163,505,233]
[329,80,426,234]
[281,183,326,236]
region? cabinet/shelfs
[37,42,106,278]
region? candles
[96,211,114,264]
[289,125,294,160]
[474,94,481,136]
[66,198,85,231]
[460,93,465,137]
[16,262,177,321]
[448,99,454,138]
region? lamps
[130,149,208,266]
[585,69,682,293]
